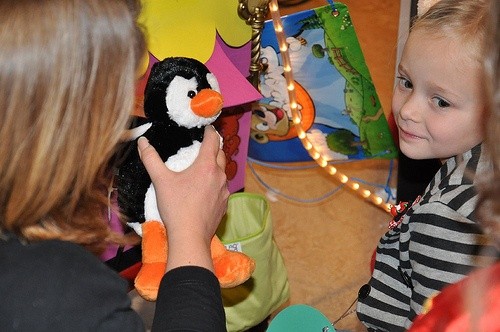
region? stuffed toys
[115,57,255,302]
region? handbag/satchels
[215,191,291,332]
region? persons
[0,0,230,332]
[395,1,446,223]
[408,0,500,332]
[356,1,500,332]
